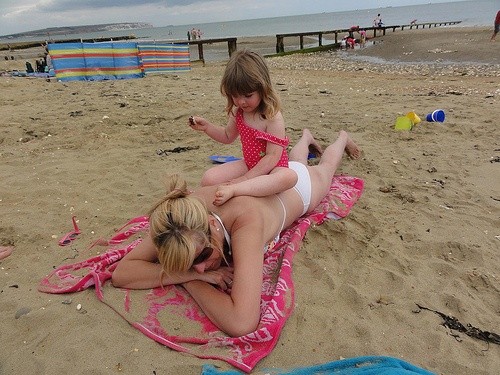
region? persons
[111,128,360,337]
[186,27,202,40]
[490,10,500,40]
[188,48,298,207]
[7,40,54,73]
[341,14,417,48]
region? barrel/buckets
[395,111,422,130]
[426,109,446,122]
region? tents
[46,41,191,82]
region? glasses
[193,222,213,265]
